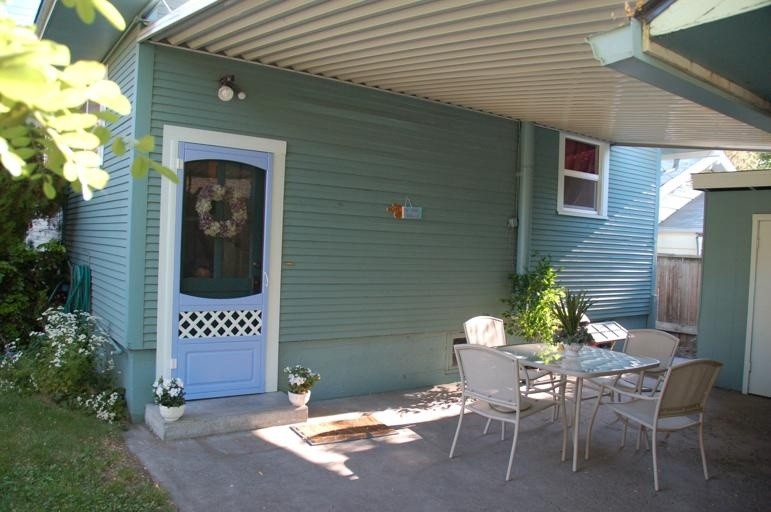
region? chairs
[449,342,570,484]
[583,357,722,493]
[573,328,681,446]
[463,316,557,440]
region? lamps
[214,71,247,102]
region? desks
[501,342,660,462]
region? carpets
[289,413,400,445]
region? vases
[287,389,311,407]
[160,406,185,423]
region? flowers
[281,360,320,393]
[151,375,186,407]
